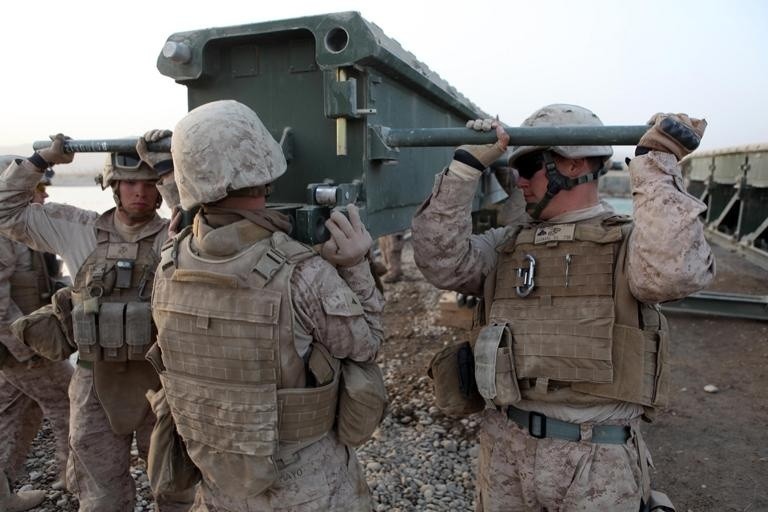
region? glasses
[516,151,543,180]
[112,152,143,171]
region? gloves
[456,118,506,168]
[41,133,74,163]
[136,129,173,176]
[639,112,707,162]
[321,204,372,267]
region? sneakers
[10,489,44,510]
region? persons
[407,102,719,512]
[0,130,210,511]
[0,154,76,511]
[378,230,407,286]
[146,96,393,510]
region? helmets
[101,150,160,188]
[0,154,52,185]
[508,104,614,167]
[172,99,287,212]
